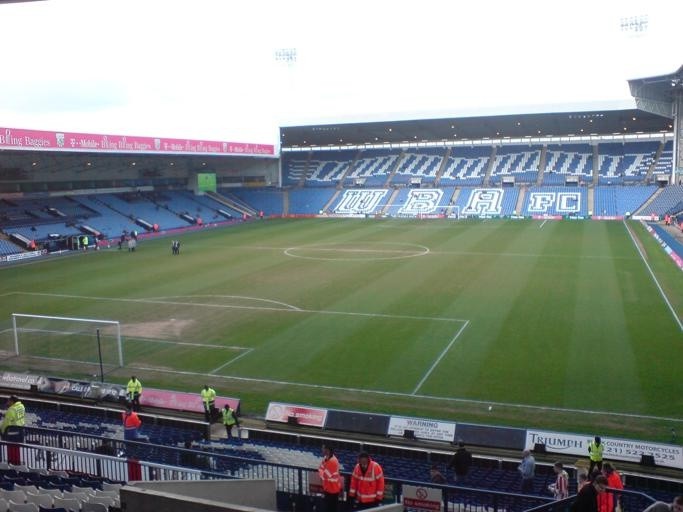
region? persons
[546,461,569,500]
[429,465,449,512]
[96,438,117,457]
[587,436,603,481]
[315,439,342,512]
[446,439,471,483]
[516,448,535,493]
[121,404,141,442]
[200,383,216,425]
[566,461,682,512]
[126,374,142,413]
[79,228,180,257]
[0,395,25,440]
[345,452,384,512]
[181,441,209,469]
[218,404,240,440]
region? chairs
[0,143,683,267]
[0,401,683,512]
